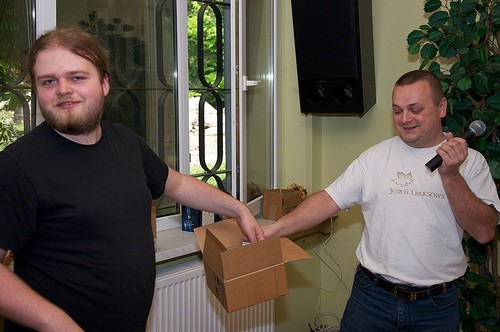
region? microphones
[425,120,486,173]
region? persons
[0,29,265,332]
[260,69,500,332]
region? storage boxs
[151,198,160,239]
[263,189,307,221]
[194,217,313,313]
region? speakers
[291,0,376,118]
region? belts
[359,266,458,301]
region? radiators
[146,258,275,332]
